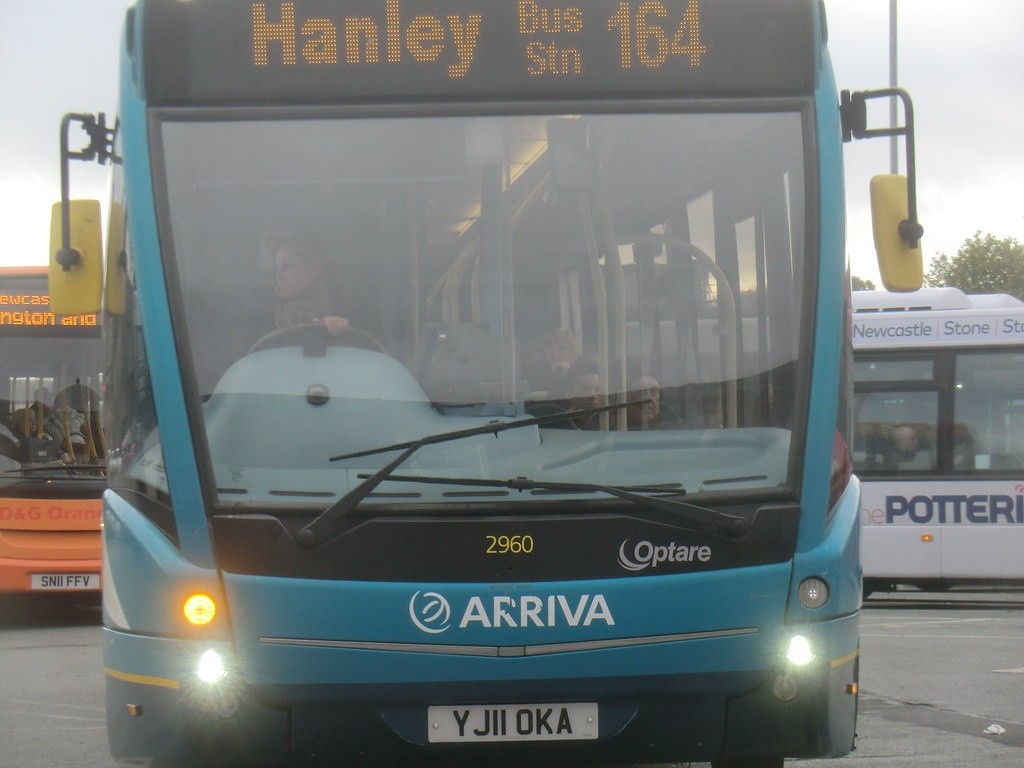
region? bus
[1,263,120,594]
[37,1,932,768]
[848,287,1024,591]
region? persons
[11,387,90,468]
[227,228,381,373]
[886,426,967,478]
[519,326,686,429]
